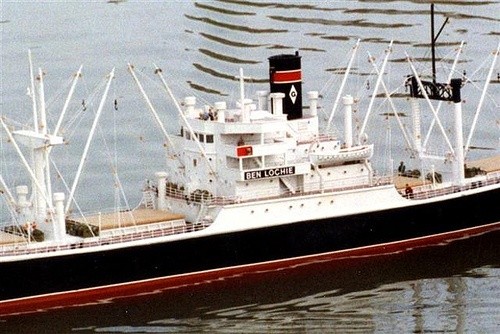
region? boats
[0,3,499,334]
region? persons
[398,161,405,176]
[405,183,414,199]
